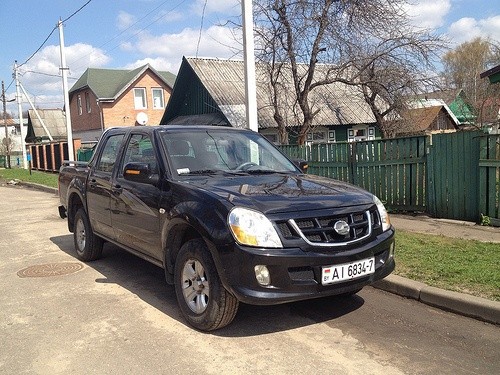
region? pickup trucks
[57,113,397,332]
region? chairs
[127,141,221,173]
